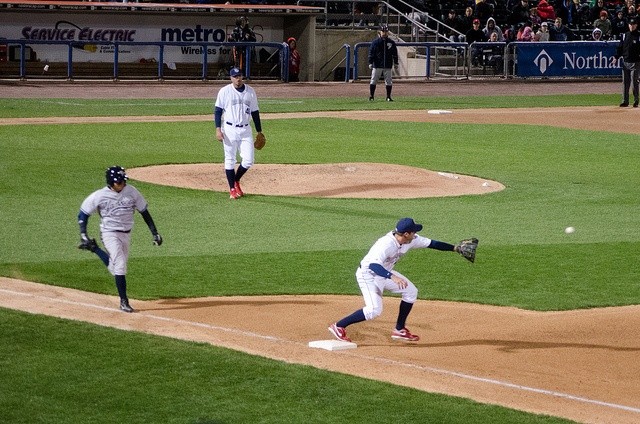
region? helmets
[107,166,129,187]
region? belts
[226,121,248,127]
[113,230,131,233]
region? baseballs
[565,226,575,234]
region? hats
[395,218,422,232]
[230,68,241,77]
[381,25,388,31]
[629,18,637,24]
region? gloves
[153,234,162,246]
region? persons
[444,9,461,29]
[619,21,640,106]
[484,18,504,41]
[553,18,579,41]
[462,7,477,32]
[594,9,611,36]
[535,2,559,20]
[614,11,628,33]
[214,68,266,199]
[579,5,593,27]
[484,34,506,73]
[465,17,485,42]
[527,8,542,24]
[368,25,400,103]
[517,22,531,42]
[502,27,515,41]
[627,4,639,24]
[327,219,462,343]
[76,165,163,312]
[591,29,605,41]
[563,0,575,23]
[537,20,551,42]
[623,0,638,13]
[592,0,608,16]
[287,38,301,81]
[514,0,530,22]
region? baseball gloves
[456,237,479,263]
[254,132,266,149]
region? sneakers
[386,97,393,102]
[120,299,133,312]
[234,181,243,196]
[78,238,98,252]
[230,188,238,199]
[328,322,352,343]
[391,328,419,341]
